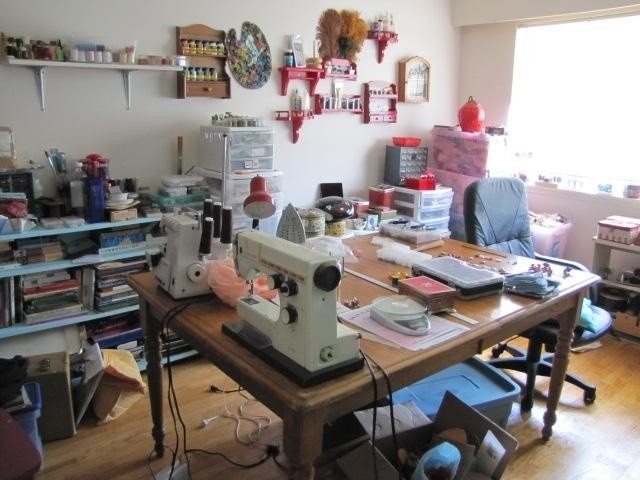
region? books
[0,223,189,362]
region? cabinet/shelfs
[0,210,164,338]
[392,184,454,240]
[591,236,640,347]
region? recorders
[312,196,354,222]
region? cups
[322,95,362,110]
[354,220,366,230]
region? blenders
[0,127,16,173]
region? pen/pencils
[476,255,492,260]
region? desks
[125,232,601,480]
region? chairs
[463,177,613,413]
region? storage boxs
[193,125,290,238]
[428,128,509,243]
[1,351,77,479]
[334,355,524,480]
[530,213,572,259]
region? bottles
[282,49,293,67]
[180,39,224,82]
[70,152,112,182]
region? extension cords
[151,461,188,480]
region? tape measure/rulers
[343,267,479,325]
